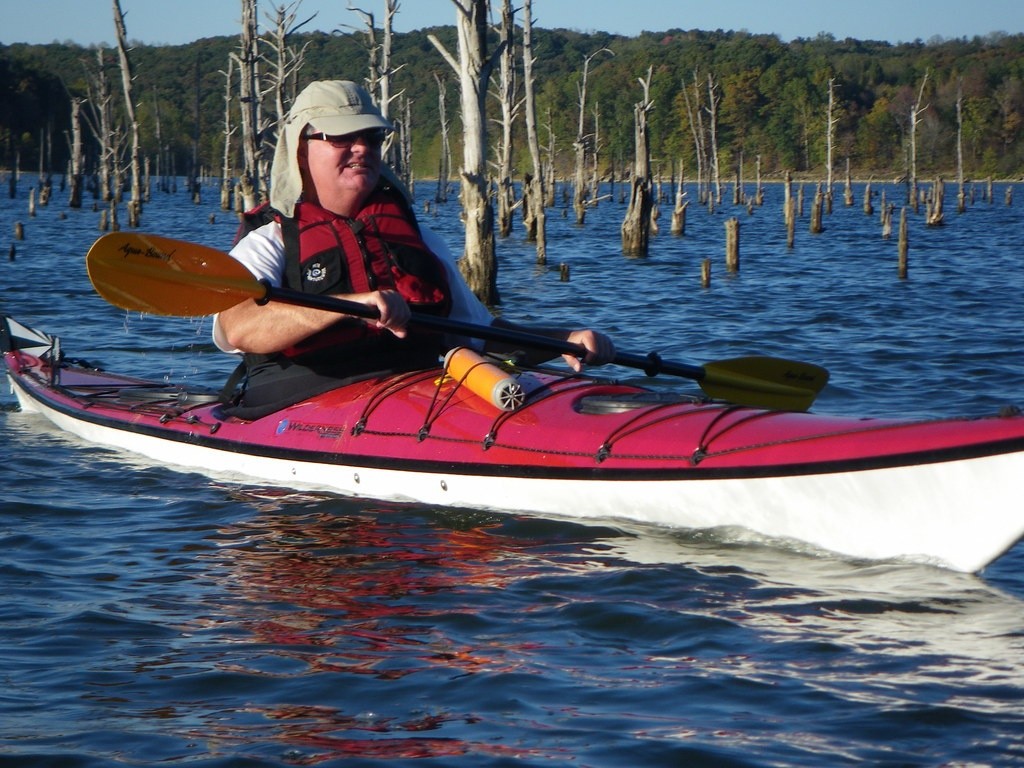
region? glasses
[301,129,385,151]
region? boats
[0,317,1024,582]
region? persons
[212,80,616,406]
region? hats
[269,79,415,219]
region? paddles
[83,229,831,415]
